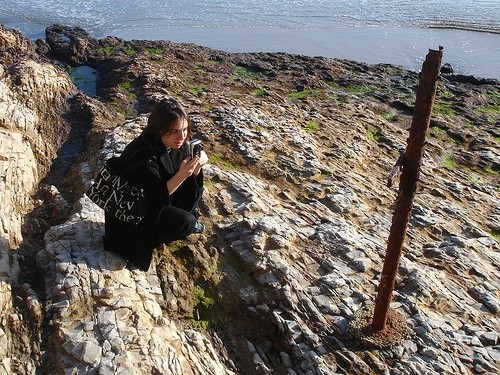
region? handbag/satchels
[86,157,160,223]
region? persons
[103,97,208,273]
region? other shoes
[193,221,205,233]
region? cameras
[189,139,203,161]
